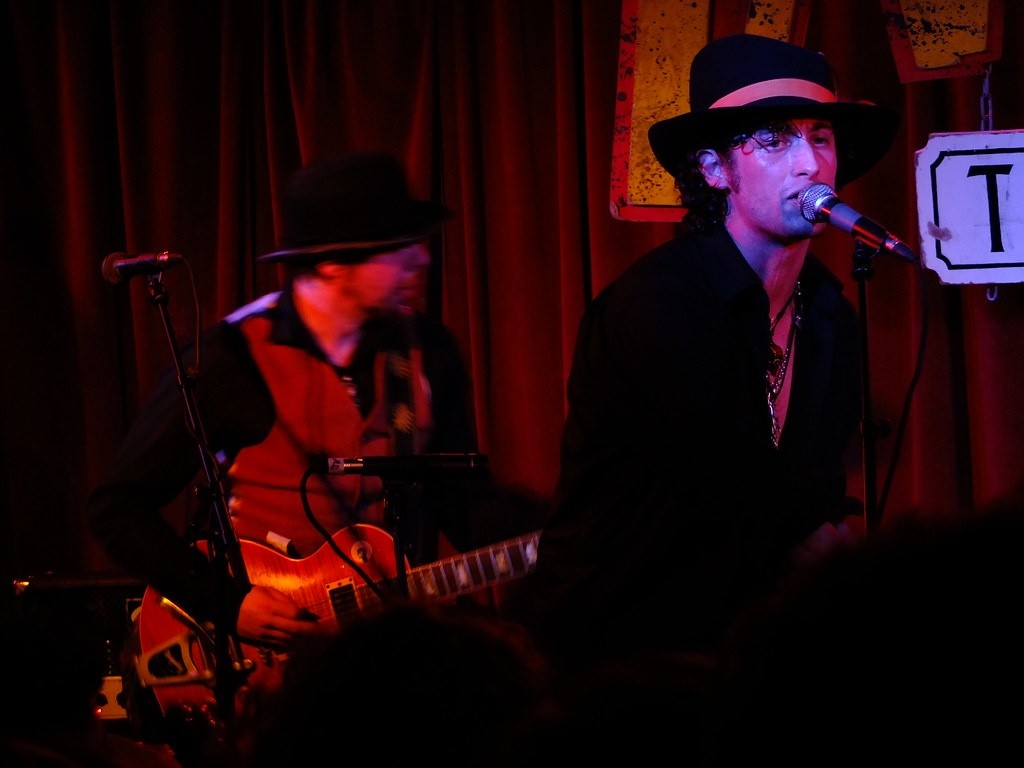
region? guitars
[121,525,545,767]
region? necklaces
[768,292,796,447]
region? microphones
[102,252,183,282]
[321,452,491,471]
[798,183,917,265]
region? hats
[257,151,452,263]
[648,34,900,190]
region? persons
[83,149,553,768]
[539,35,890,674]
